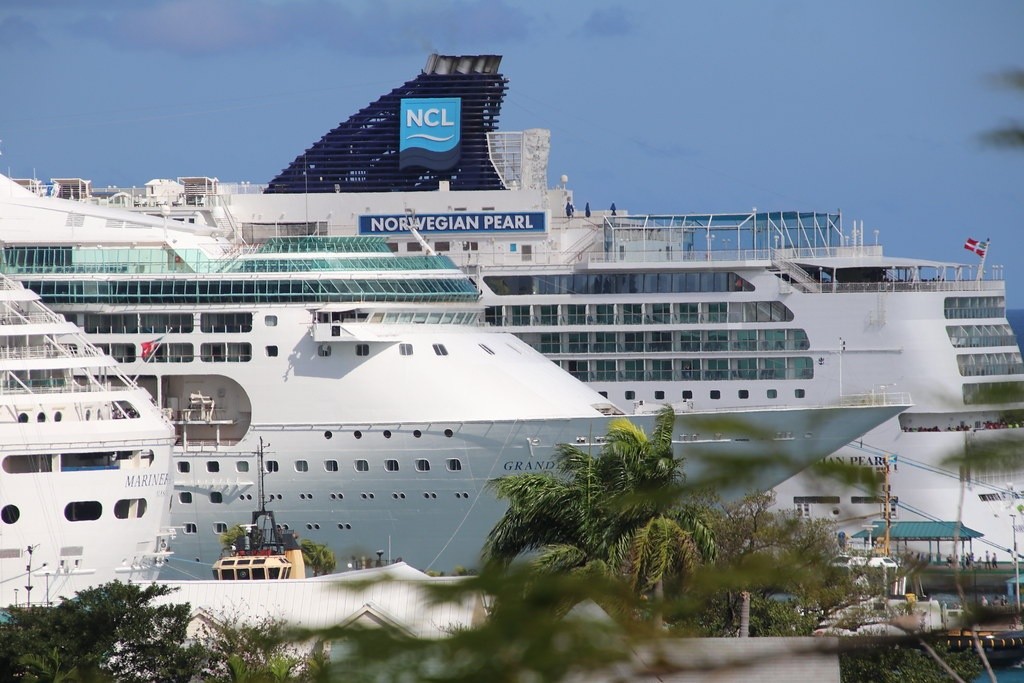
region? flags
[963,236,988,258]
[140,336,165,359]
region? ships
[0,172,1024,629]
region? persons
[903,417,1024,432]
[910,550,999,570]
[942,594,1009,610]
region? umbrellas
[567,203,574,216]
[586,203,591,217]
[610,201,616,216]
